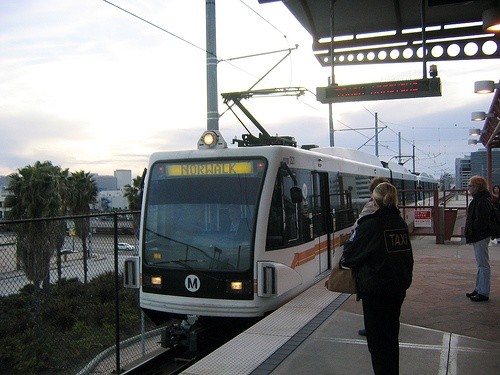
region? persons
[340,181,414,375]
[492,185,500,206]
[229,205,248,241]
[464,176,493,301]
[351,177,390,335]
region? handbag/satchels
[325,255,357,294]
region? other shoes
[466,290,478,298]
[470,293,489,302]
[359,330,366,336]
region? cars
[118,243,136,251]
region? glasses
[469,185,473,187]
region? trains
[138,86,442,352]
